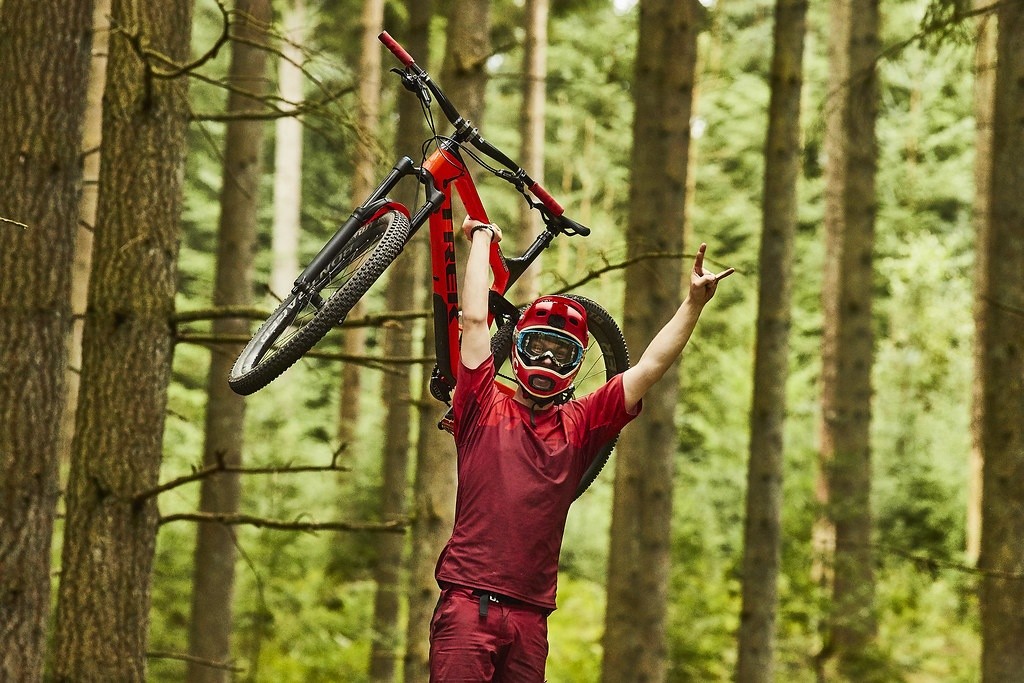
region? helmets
[511,295,588,399]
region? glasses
[517,330,582,368]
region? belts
[473,588,516,616]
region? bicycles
[227,25,632,506]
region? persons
[426,212,734,683]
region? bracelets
[470,224,495,242]
